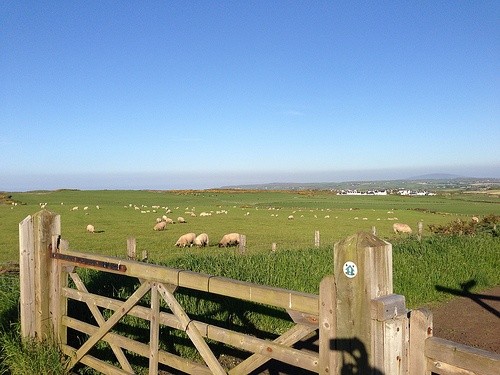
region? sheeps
[122,203,161,214]
[176,216,186,224]
[386,208,394,214]
[267,207,280,216]
[349,207,353,211]
[218,205,222,208]
[391,222,414,235]
[199,209,229,217]
[86,224,95,233]
[72,206,79,212]
[175,207,180,210]
[309,208,332,219]
[153,214,174,231]
[288,209,305,220]
[95,205,100,210]
[162,206,171,214]
[60,202,65,205]
[184,206,197,217]
[255,207,258,210]
[39,202,48,209]
[376,217,399,221]
[232,205,237,209]
[370,208,376,212]
[174,231,210,248]
[353,216,368,221]
[241,208,250,216]
[83,206,89,211]
[335,216,338,219]
[218,232,241,247]
[10,202,18,209]
